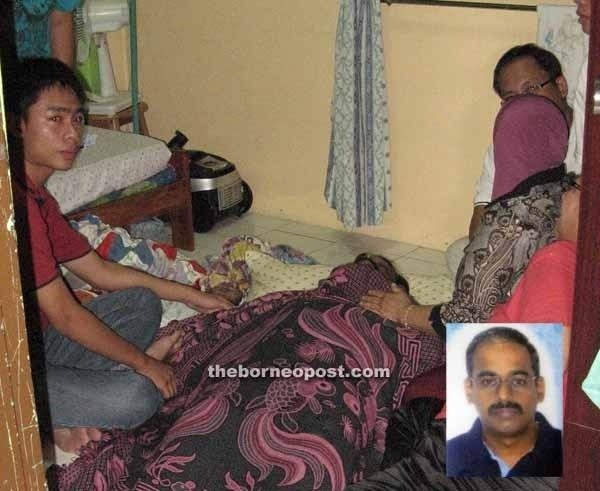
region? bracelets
[403,303,417,330]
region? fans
[74,1,143,117]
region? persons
[7,0,87,69]
[356,95,571,343]
[563,1,593,174]
[4,55,235,454]
[444,327,564,477]
[467,42,575,244]
[406,173,582,430]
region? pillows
[240,248,456,318]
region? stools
[86,98,153,136]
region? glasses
[499,78,557,106]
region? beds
[43,122,200,253]
